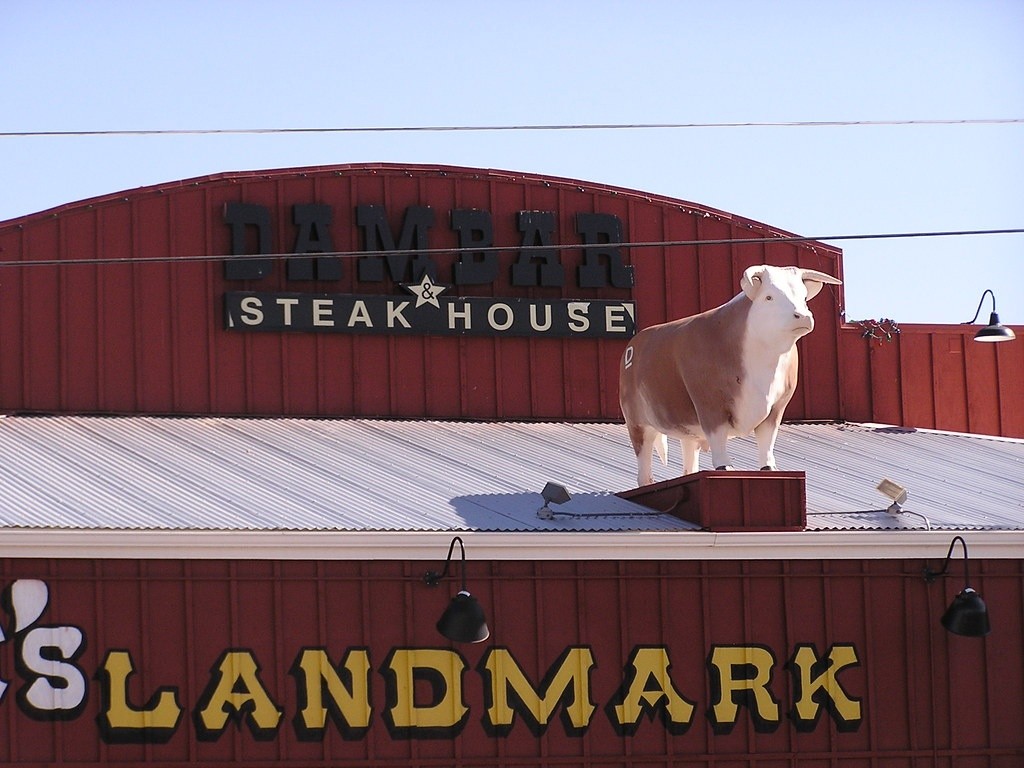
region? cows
[616,263,843,487]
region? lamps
[924,535,994,637]
[875,478,909,515]
[535,481,572,519]
[960,289,1016,342]
[424,536,489,643]
